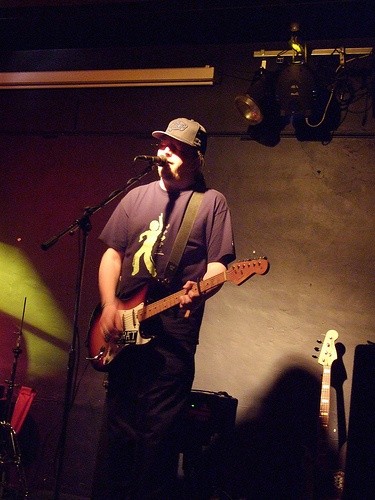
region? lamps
[231,68,276,128]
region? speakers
[162,392,238,500]
[342,344,375,500]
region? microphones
[136,154,167,166]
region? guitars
[313,328,345,500]
[86,256,271,373]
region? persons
[96,117,235,500]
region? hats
[152,118,207,154]
